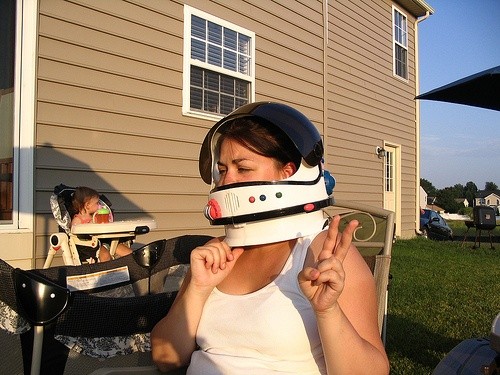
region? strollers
[42,184,157,267]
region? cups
[92,207,110,223]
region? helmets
[199,101,335,246]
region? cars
[421,208,454,242]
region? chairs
[90,199,394,375]
[43,184,157,269]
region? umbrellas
[412,65,499,110]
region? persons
[150,101,389,375]
[433,311,500,375]
[70,187,136,265]
